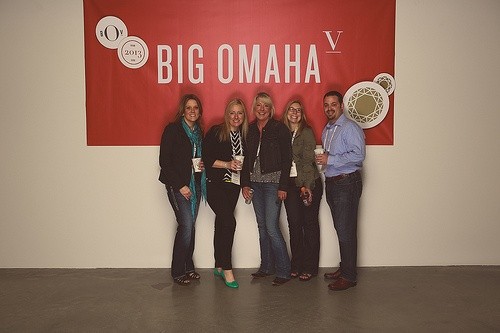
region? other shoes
[271,278,288,285]
[251,271,269,277]
[328,278,356,290]
[324,268,341,278]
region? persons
[240,93,291,285]
[158,95,207,285]
[316,91,365,291]
[282,100,323,280]
[202,98,249,288]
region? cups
[245,189,254,204]
[235,156,244,170]
[192,158,202,172]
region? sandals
[174,275,190,285]
[187,272,201,279]
[298,273,312,280]
[291,272,299,278]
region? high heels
[214,268,221,275]
[221,270,239,288]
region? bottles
[314,144,324,166]
[300,187,312,207]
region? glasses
[289,107,302,113]
